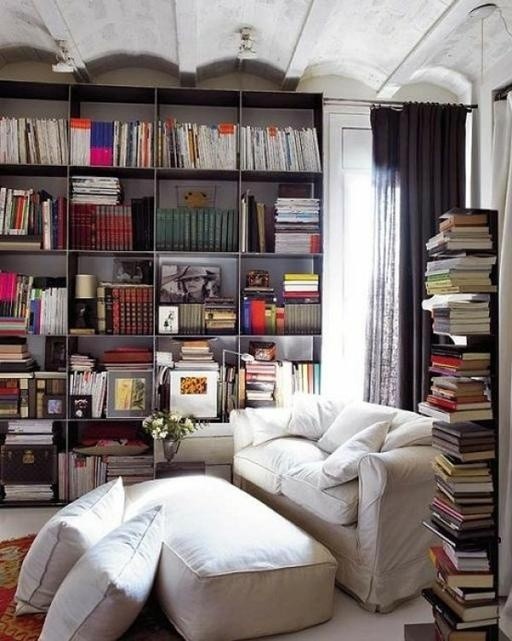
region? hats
[174,265,216,282]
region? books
[416,213,501,641]
[0,334,322,420]
[0,116,321,172]
[1,251,322,334]
[1,419,153,503]
[1,175,322,254]
